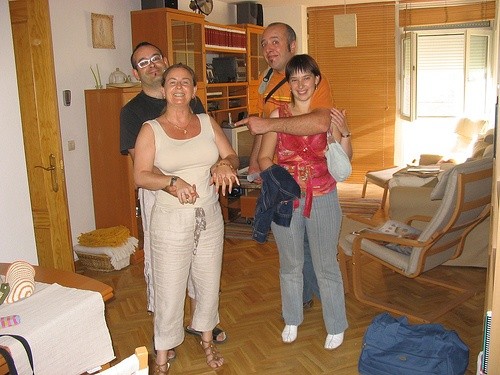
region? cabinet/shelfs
[83,8,274,266]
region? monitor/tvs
[220,125,255,175]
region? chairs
[361,117,489,210]
[338,157,493,324]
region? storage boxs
[75,251,131,273]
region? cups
[108,67,127,84]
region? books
[205,26,247,50]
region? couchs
[389,128,495,267]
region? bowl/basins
[441,162,455,171]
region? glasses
[135,54,163,69]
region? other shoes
[282,325,298,343]
[324,331,344,350]
[302,300,314,309]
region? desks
[0,263,114,375]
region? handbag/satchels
[324,129,352,182]
[358,313,470,375]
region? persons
[235,22,334,318]
[257,54,353,351]
[132,62,241,375]
[120,41,227,360]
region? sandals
[199,339,225,369]
[154,356,170,375]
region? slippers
[185,324,227,343]
[152,336,176,359]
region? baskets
[75,251,134,272]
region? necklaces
[165,114,193,133]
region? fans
[189,0,213,22]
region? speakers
[140,0,178,10]
[236,3,263,26]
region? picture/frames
[91,13,116,49]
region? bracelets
[220,162,233,169]
[170,175,180,186]
[341,133,351,137]
